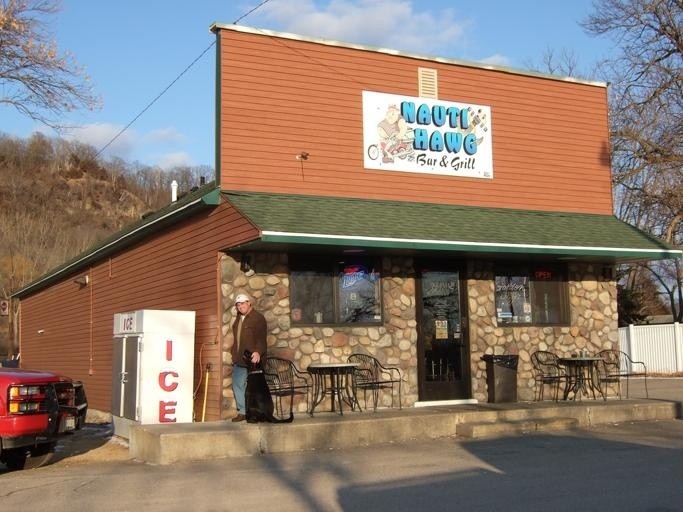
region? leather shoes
[232,414,246,422]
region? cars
[0,367,88,467]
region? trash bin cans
[484,355,520,403]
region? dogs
[240,349,294,424]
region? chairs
[262,356,314,418]
[592,348,649,400]
[344,352,401,409]
[530,350,568,402]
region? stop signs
[1,301,8,312]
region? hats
[234,294,250,305]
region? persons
[231,294,267,422]
